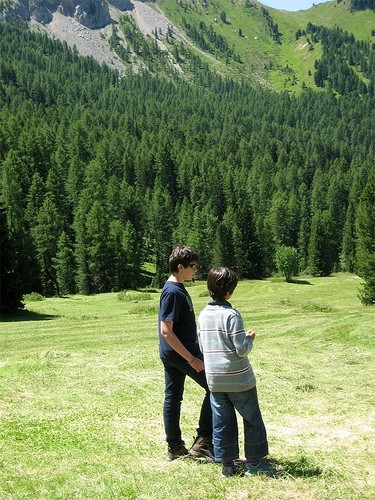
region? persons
[159,245,216,462]
[196,266,274,479]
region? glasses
[186,263,196,269]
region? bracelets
[188,356,195,365]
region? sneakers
[165,445,187,461]
[243,460,276,478]
[219,461,236,477]
[188,435,214,460]
